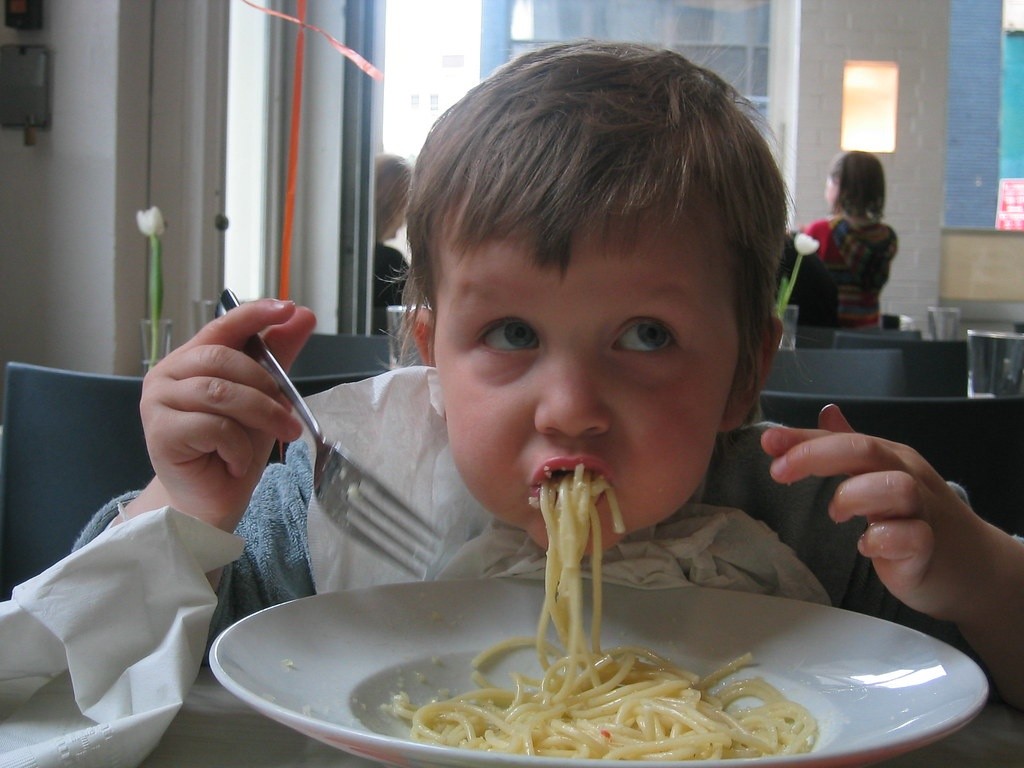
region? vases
[926,307,963,341]
[140,321,171,374]
[778,305,800,351]
[966,329,1023,400]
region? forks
[212,288,442,577]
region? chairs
[759,326,1023,530]
[0,333,394,601]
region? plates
[207,578,988,768]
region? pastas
[397,462,817,759]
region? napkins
[0,507,245,768]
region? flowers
[775,232,821,313]
[136,207,164,370]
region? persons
[76,38,1024,712]
[371,152,414,334]
[775,148,897,332]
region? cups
[776,305,798,351]
[138,318,175,377]
[385,305,433,369]
[967,328,1024,400]
[927,307,963,341]
[879,299,901,331]
